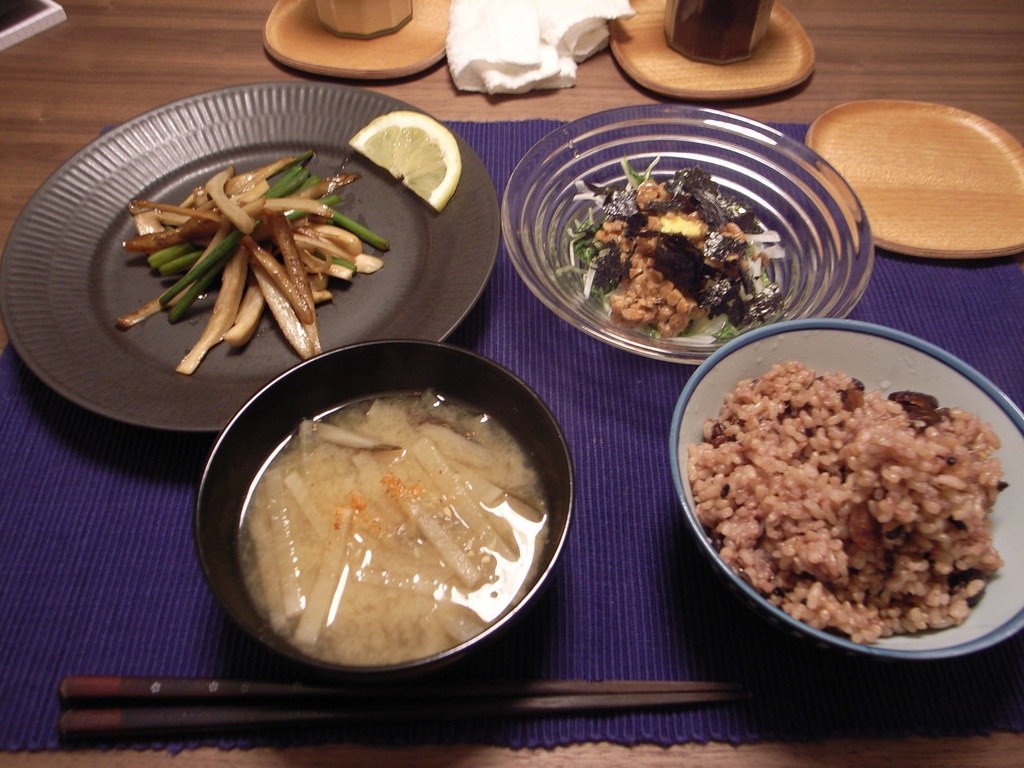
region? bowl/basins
[195,340,577,676]
[499,103,874,365]
[668,318,1024,659]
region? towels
[444,0,635,95]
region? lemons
[349,110,462,213]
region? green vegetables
[568,154,740,347]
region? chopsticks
[61,674,755,733]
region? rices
[686,360,1005,646]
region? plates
[806,101,1024,259]
[608,0,816,99]
[0,82,499,431]
[262,0,451,79]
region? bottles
[316,0,412,39]
[664,0,775,64]
